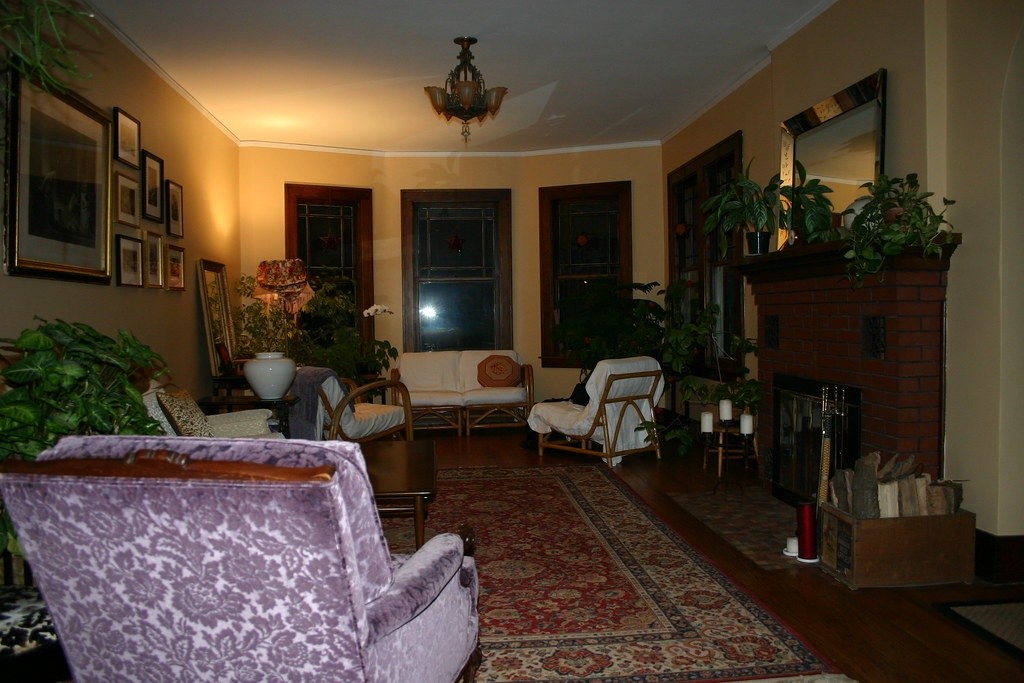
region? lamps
[423,35,513,142]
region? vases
[359,374,379,383]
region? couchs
[0,433,479,683]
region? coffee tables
[346,439,436,554]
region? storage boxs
[820,506,976,590]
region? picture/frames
[198,258,234,377]
[11,72,184,289]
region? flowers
[358,304,398,380]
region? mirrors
[774,69,887,251]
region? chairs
[295,366,413,443]
[526,355,665,470]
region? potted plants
[238,270,361,395]
[705,159,956,277]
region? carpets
[379,459,854,683]
[931,598,1024,662]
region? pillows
[477,354,523,386]
[154,387,212,441]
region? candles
[740,413,753,435]
[700,411,713,434]
[719,399,732,420]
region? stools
[703,420,761,478]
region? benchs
[389,350,533,442]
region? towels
[585,357,667,470]
[285,366,353,442]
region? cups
[785,536,798,553]
